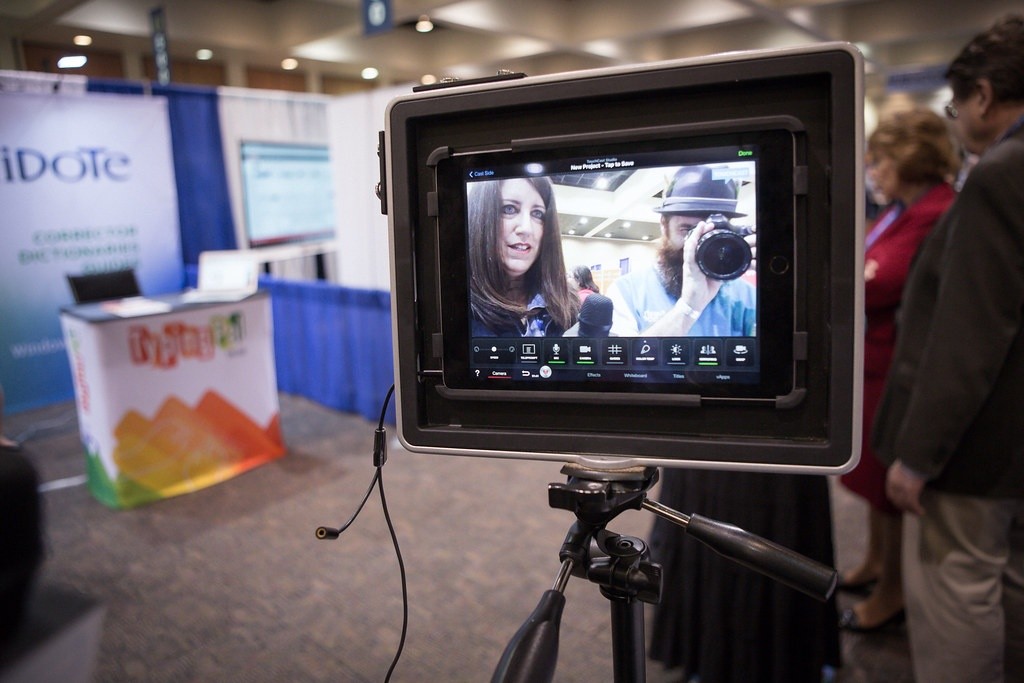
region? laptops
[178,250,259,303]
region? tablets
[429,117,806,411]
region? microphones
[578,293,613,338]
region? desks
[57,290,288,509]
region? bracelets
[676,299,700,320]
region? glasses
[943,85,974,119]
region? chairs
[196,249,259,288]
[65,270,140,306]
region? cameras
[694,213,753,279]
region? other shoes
[839,566,883,594]
[838,599,906,632]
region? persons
[605,158,757,336]
[647,14,1024,683]
[467,174,578,338]
[567,264,602,304]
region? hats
[653,165,748,219]
